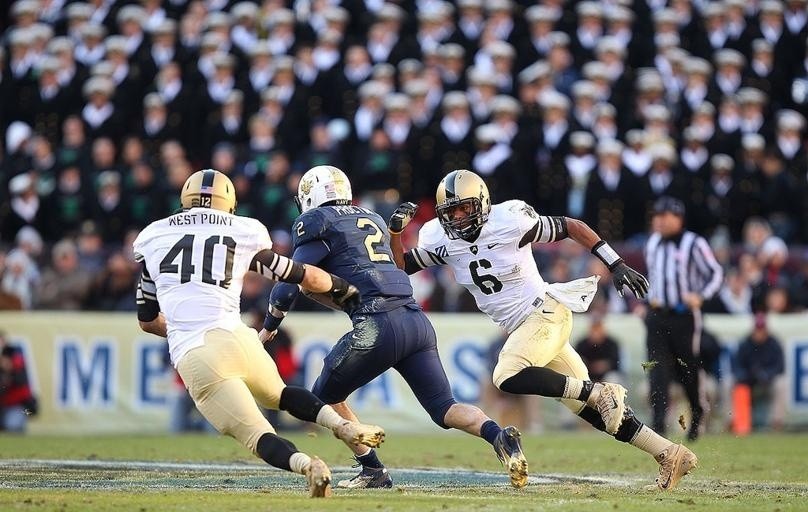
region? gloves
[388,202,418,234]
[608,260,648,299]
[325,274,362,312]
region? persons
[0,327,38,434]
[0,1,808,313]
[163,334,215,434]
[697,320,723,439]
[388,168,698,490]
[132,168,386,498]
[487,329,539,435]
[246,298,299,429]
[576,322,626,431]
[255,165,527,490]
[731,308,792,434]
[642,193,724,442]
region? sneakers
[335,419,385,448]
[645,443,698,491]
[493,426,529,488]
[305,456,333,499]
[337,465,394,489]
[587,382,629,435]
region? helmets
[181,169,237,215]
[295,165,353,214]
[436,170,490,240]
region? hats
[650,197,684,216]
